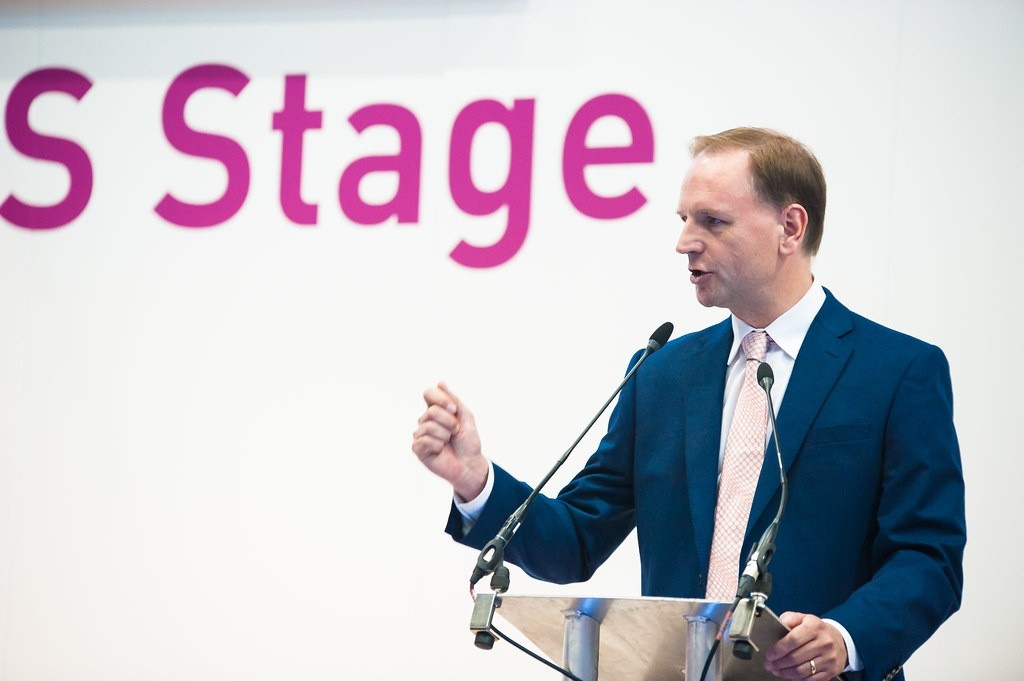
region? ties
[705,329,774,603]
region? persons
[412,126,966,681]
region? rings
[810,660,816,675]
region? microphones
[469,320,676,589]
[736,363,789,597]
